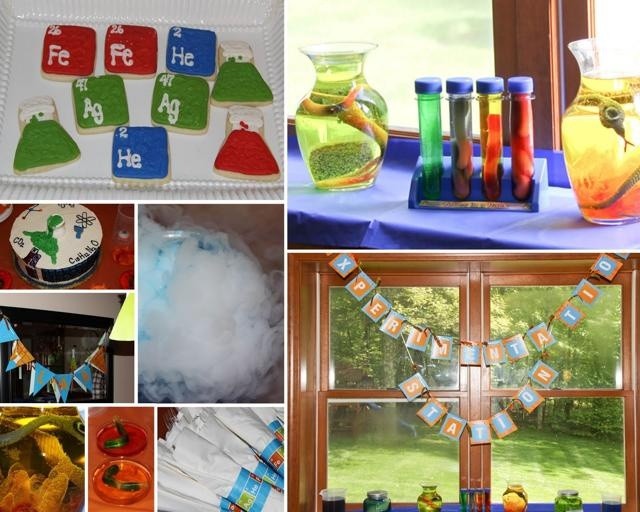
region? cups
[319,487,348,512]
[601,495,623,512]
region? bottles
[444,77,476,200]
[417,483,442,512]
[460,488,491,512]
[23,344,85,398]
[413,77,445,201]
[507,75,536,204]
[559,36,640,227]
[555,489,583,512]
[475,76,506,200]
[363,490,391,512]
[503,478,528,512]
[294,37,390,192]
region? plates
[96,421,149,457]
[91,459,153,505]
